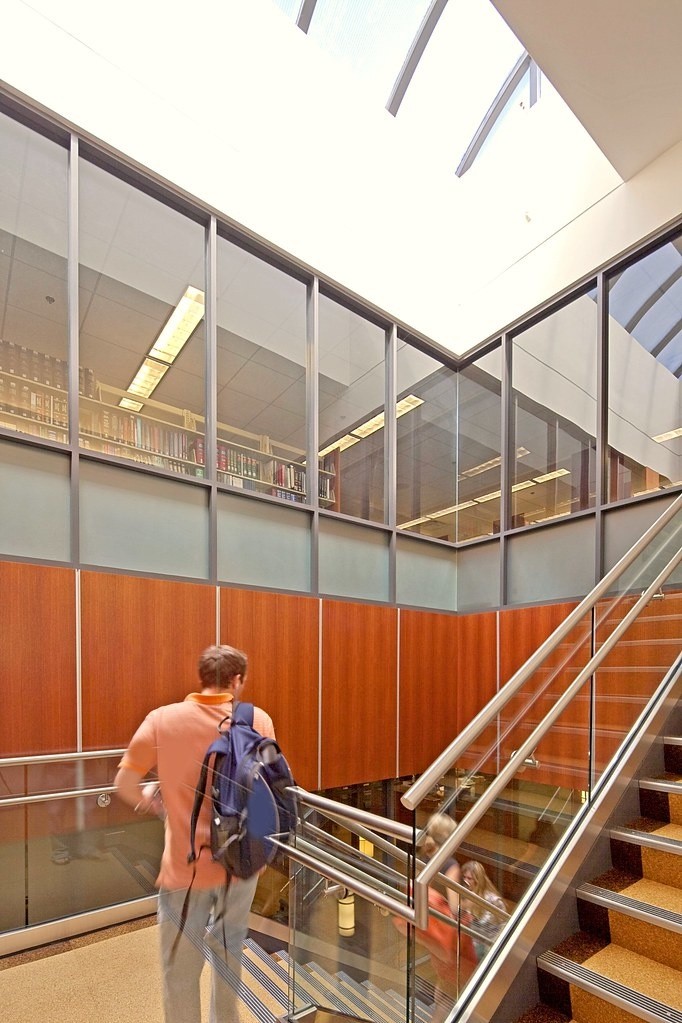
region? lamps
[338,887,355,938]
[359,835,374,859]
[301,433,362,465]
[348,393,426,439]
[146,284,205,365]
[117,356,171,413]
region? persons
[42,760,109,865]
[460,860,512,966]
[111,644,276,1022]
[406,813,479,1023]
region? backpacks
[187,701,298,879]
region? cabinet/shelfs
[0,371,341,512]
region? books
[1,339,337,509]
